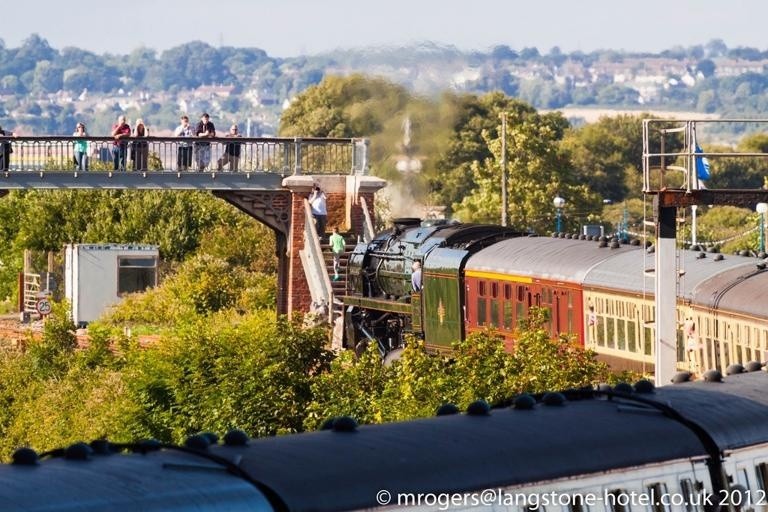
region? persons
[0,125,14,178]
[216,124,244,172]
[407,260,422,297]
[112,113,131,172]
[71,121,91,172]
[309,187,327,241]
[132,117,150,172]
[308,186,328,225]
[328,226,347,282]
[193,113,216,173]
[173,114,193,176]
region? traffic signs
[36,299,52,314]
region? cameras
[316,187,320,191]
[123,124,129,128]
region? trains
[0,197,768,510]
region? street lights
[396,118,423,215]
